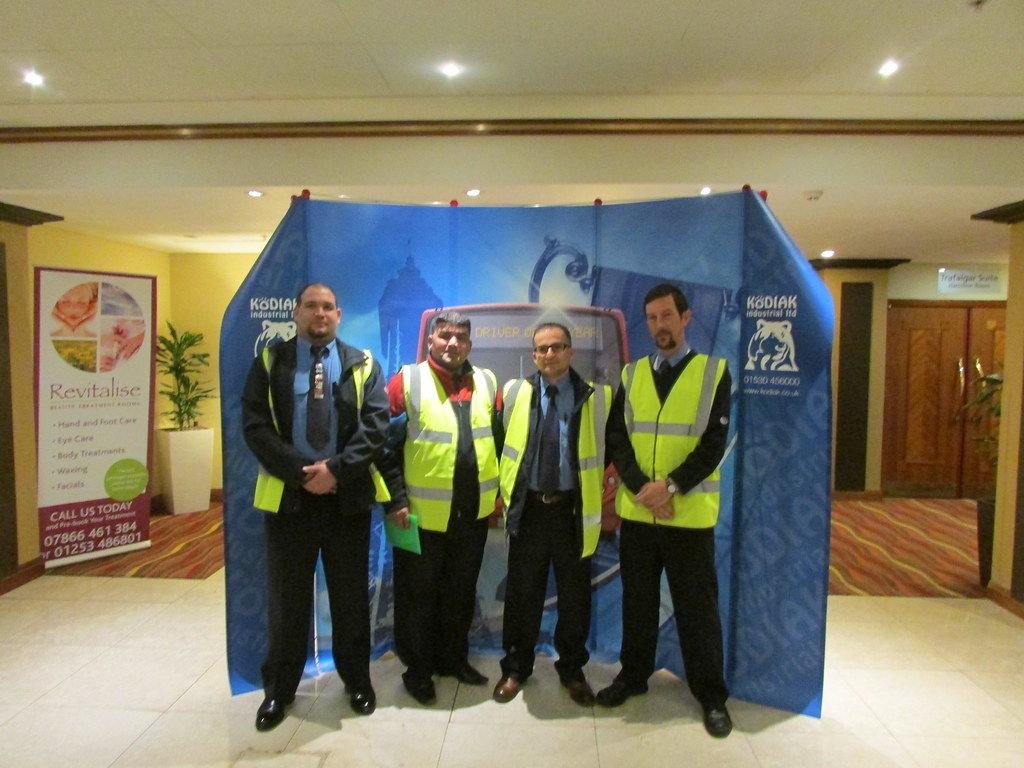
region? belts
[450,507,477,519]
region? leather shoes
[256,688,297,731]
[493,672,527,702]
[559,671,595,706]
[344,679,377,713]
[703,702,732,737]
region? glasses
[532,341,571,354]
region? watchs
[665,478,676,494]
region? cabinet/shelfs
[881,299,1007,500]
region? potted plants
[155,320,220,517]
[961,359,1004,589]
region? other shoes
[438,657,490,685]
[401,666,437,706]
[595,672,650,707]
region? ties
[307,345,332,451]
[527,489,575,505]
[537,386,562,492]
[659,360,670,375]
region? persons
[597,284,732,739]
[241,284,391,732]
[493,323,614,707]
[379,312,497,705]
